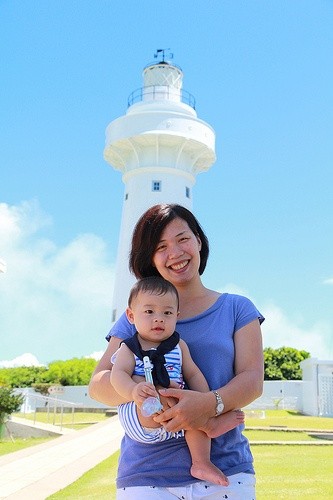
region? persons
[88,203,266,499]
[110,277,244,487]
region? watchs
[213,390,224,417]
